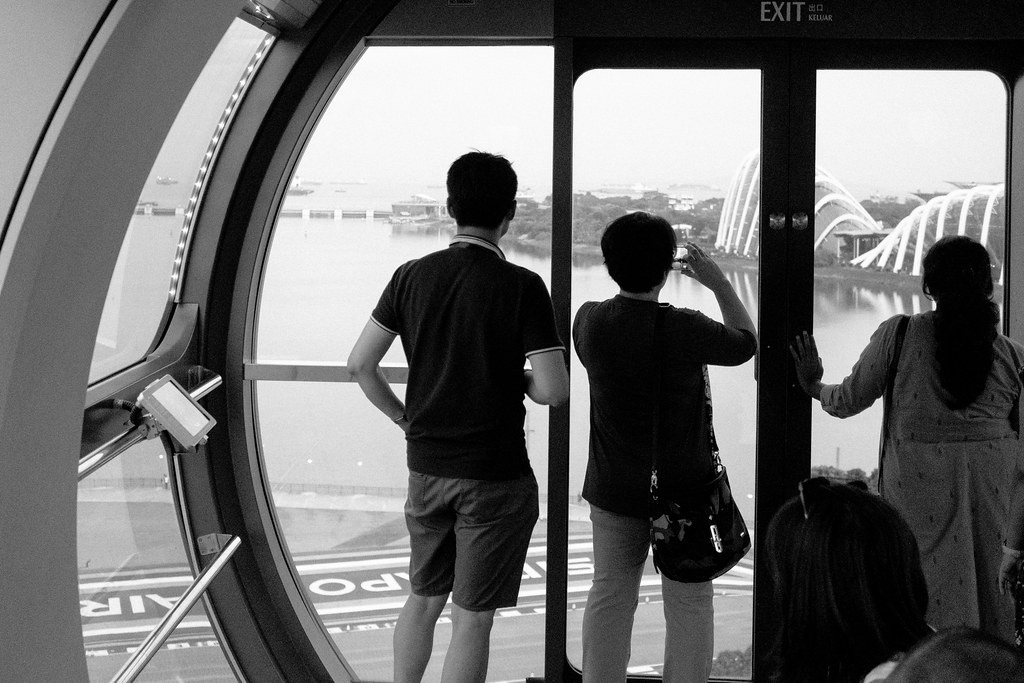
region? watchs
[393,414,407,424]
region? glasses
[797,476,869,521]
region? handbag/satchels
[647,462,751,583]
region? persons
[767,236,1024,683]
[346,152,570,683]
[572,212,758,683]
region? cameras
[671,246,690,271]
